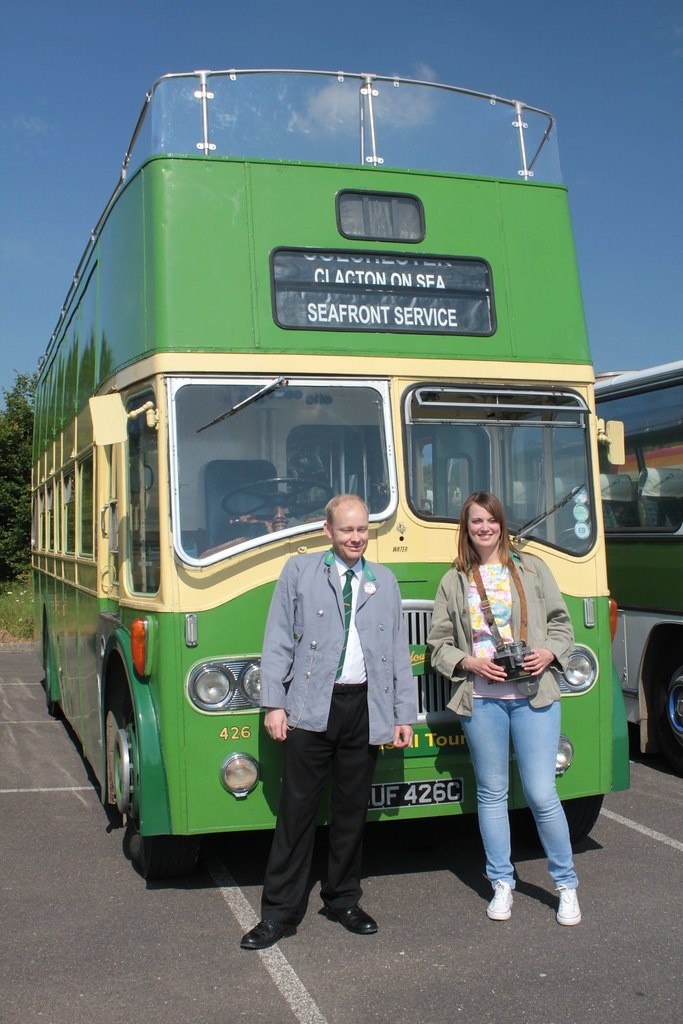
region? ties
[335,570,355,681]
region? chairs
[600,468,683,528]
[514,480,538,521]
[203,460,279,548]
[556,478,578,500]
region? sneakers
[555,883,582,926]
[486,878,514,921]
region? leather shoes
[240,918,297,949]
[327,902,379,934]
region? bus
[442,359,683,779]
[31,66,633,881]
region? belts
[333,680,368,694]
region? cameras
[492,640,535,682]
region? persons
[240,495,418,950]
[427,491,582,925]
